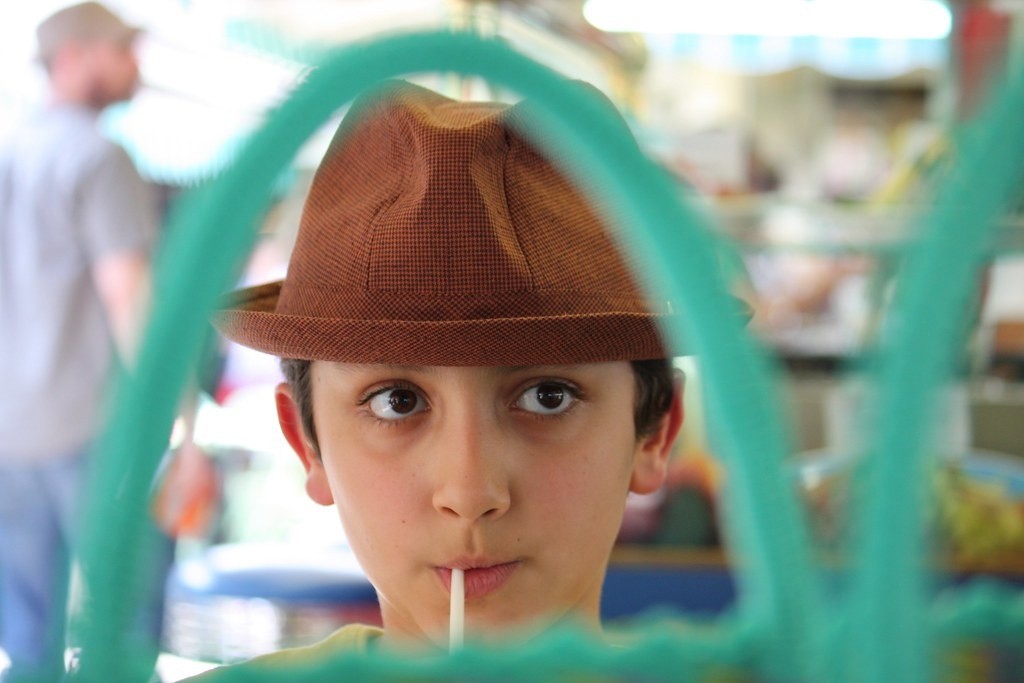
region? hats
[207,78,755,366]
[28,1,140,69]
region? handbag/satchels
[149,395,223,541]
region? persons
[0,0,198,683]
[204,74,755,683]
